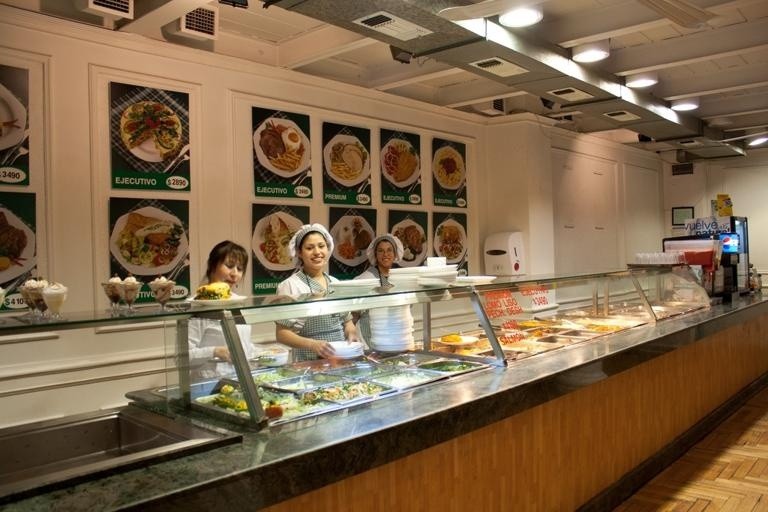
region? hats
[366,233,405,267]
[287,222,335,266]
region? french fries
[329,162,359,180]
[269,144,301,172]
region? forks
[171,154,189,173]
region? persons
[274,223,362,363]
[174,240,265,380]
[349,233,405,350]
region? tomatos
[265,404,283,419]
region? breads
[442,334,463,342]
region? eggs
[282,127,300,150]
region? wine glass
[15,280,67,322]
[100,278,141,314]
[149,282,180,313]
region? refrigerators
[663,216,749,297]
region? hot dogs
[195,282,232,301]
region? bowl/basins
[255,348,289,368]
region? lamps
[497,1,546,29]
[570,38,609,63]
[671,97,700,112]
[626,69,659,89]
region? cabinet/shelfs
[180,266,710,422]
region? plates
[328,277,381,295]
[390,220,427,268]
[109,206,186,277]
[367,302,416,354]
[0,83,28,150]
[0,205,36,286]
[129,137,162,164]
[437,336,478,346]
[323,133,370,187]
[321,341,365,361]
[384,264,497,286]
[433,217,467,265]
[252,117,311,180]
[251,211,305,272]
[432,147,466,191]
[381,137,420,189]
[329,214,375,268]
[190,295,253,306]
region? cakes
[18,277,67,315]
[102,273,141,305]
[148,274,174,303]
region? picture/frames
[671,206,695,226]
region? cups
[634,249,687,265]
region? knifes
[0,127,30,167]
[162,143,190,173]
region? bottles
[748,263,762,294]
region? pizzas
[119,102,182,161]
